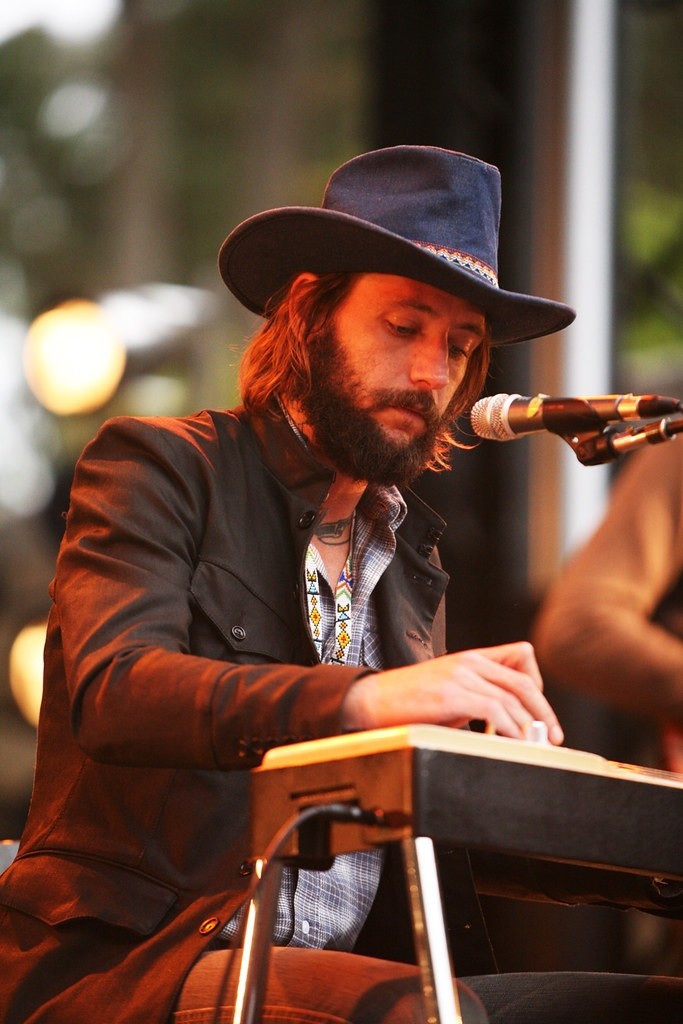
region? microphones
[470,392,683,441]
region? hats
[219,146,575,349]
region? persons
[1,145,683,1024]
[534,397,683,776]
